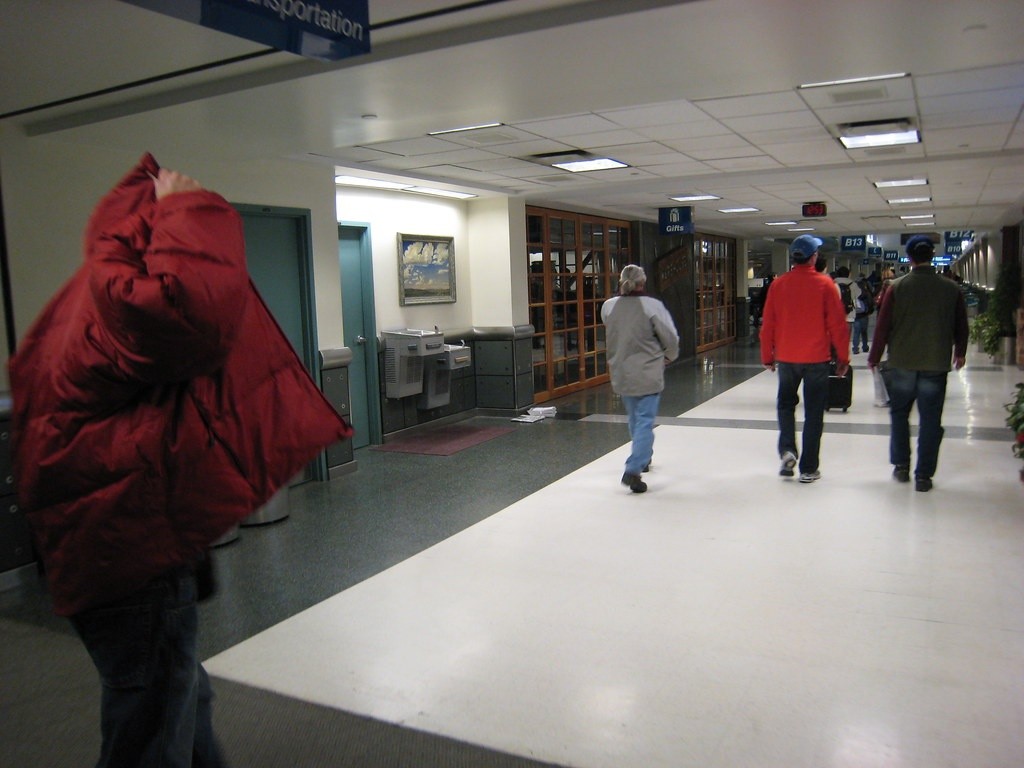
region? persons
[817,260,963,377]
[749,266,773,327]
[760,235,851,483]
[869,233,969,492]
[601,264,679,494]
[8,150,356,768]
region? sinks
[381,328,444,358]
[444,343,470,352]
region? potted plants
[969,265,1015,365]
[1003,383,1024,459]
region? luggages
[824,360,853,412]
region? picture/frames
[397,233,456,305]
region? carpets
[369,427,517,456]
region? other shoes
[622,473,647,492]
[893,465,909,481]
[800,468,821,483]
[914,475,931,492]
[779,452,798,477]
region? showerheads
[460,339,465,347]
[434,324,439,333]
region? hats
[789,235,823,261]
[906,234,935,255]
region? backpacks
[856,300,866,314]
[838,281,854,314]
